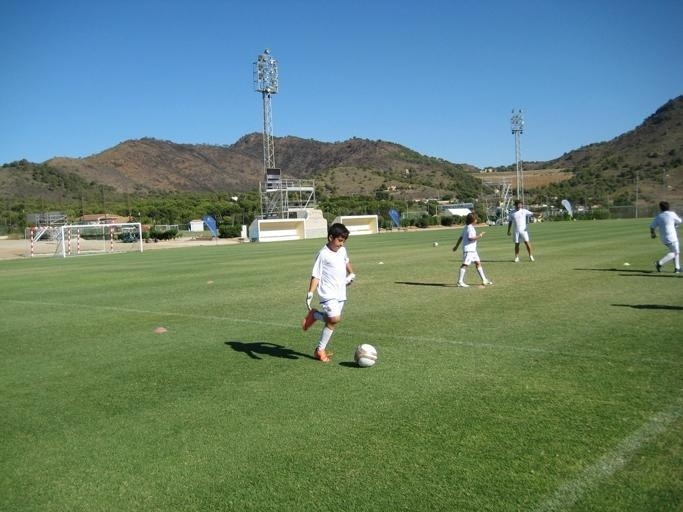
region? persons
[451,212,493,288]
[649,200,683,273]
[299,223,356,362]
[506,200,543,263]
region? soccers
[432,241,438,247]
[354,344,378,366]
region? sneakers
[655,261,661,271]
[302,308,318,330]
[314,347,330,362]
[457,282,469,287]
[483,281,492,285]
[674,268,683,273]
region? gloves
[306,292,313,311]
[346,273,355,285]
